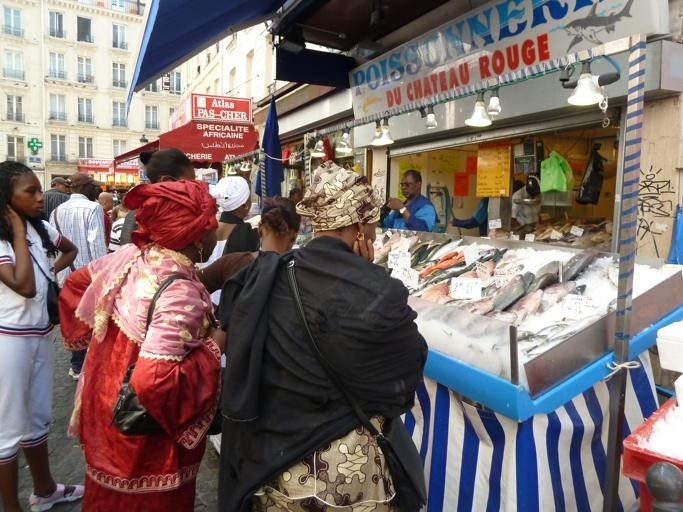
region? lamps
[305,51,626,161]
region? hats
[71,173,105,187]
[50,177,70,187]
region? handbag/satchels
[112,274,225,436]
[46,279,65,325]
[379,414,428,512]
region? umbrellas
[255,96,284,207]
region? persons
[220,161,431,512]
[450,172,544,239]
[42,147,301,380]
[1,160,85,511]
[380,169,437,233]
[57,180,227,512]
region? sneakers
[28,483,86,510]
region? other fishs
[372,232,598,356]
